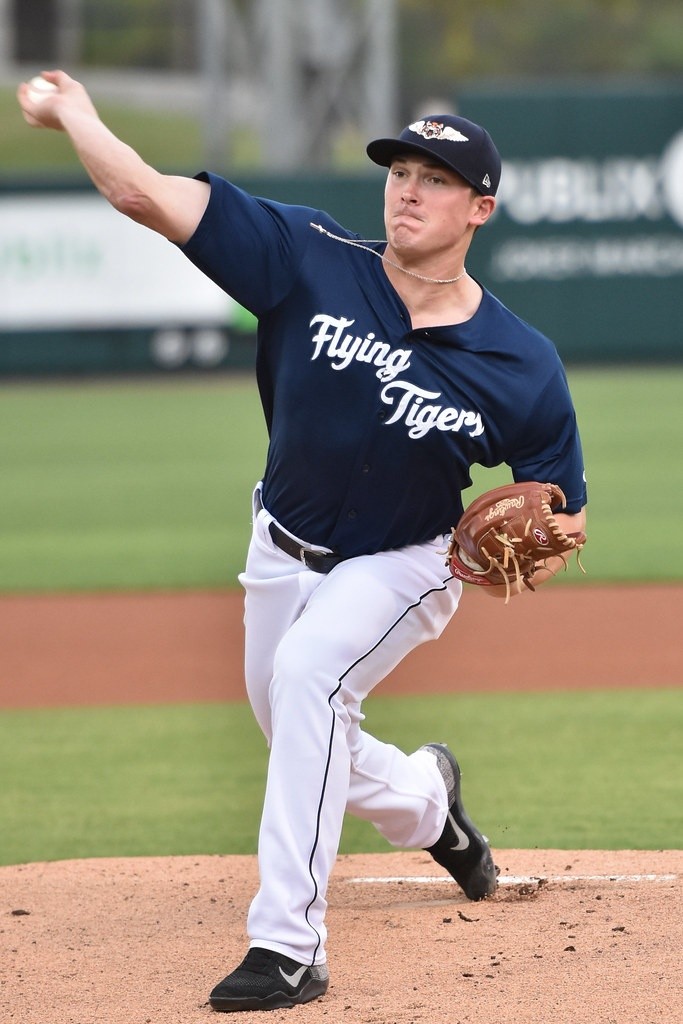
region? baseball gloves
[444,480,587,587]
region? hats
[366,113,501,198]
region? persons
[19,67,587,1008]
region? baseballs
[26,76,59,103]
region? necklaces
[308,220,465,287]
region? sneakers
[208,947,329,1012]
[419,743,500,901]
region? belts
[254,478,345,574]
